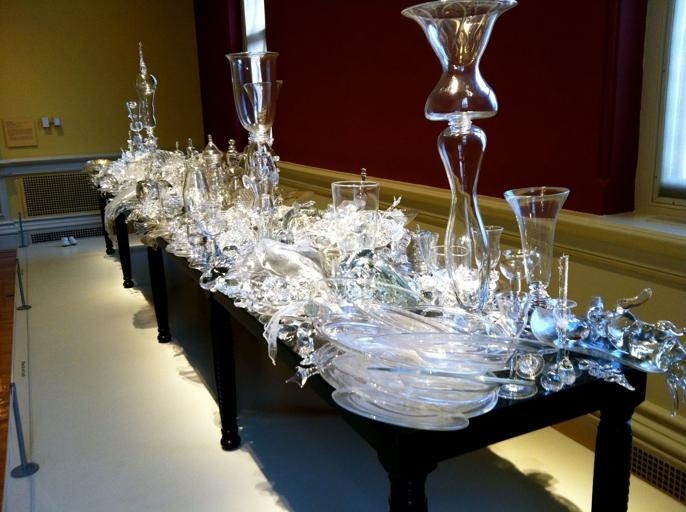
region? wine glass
[498,247,542,302]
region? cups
[330,180,379,212]
[471,224,504,271]
[428,244,471,272]
[503,185,572,302]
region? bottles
[180,137,243,207]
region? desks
[93,185,649,510]
